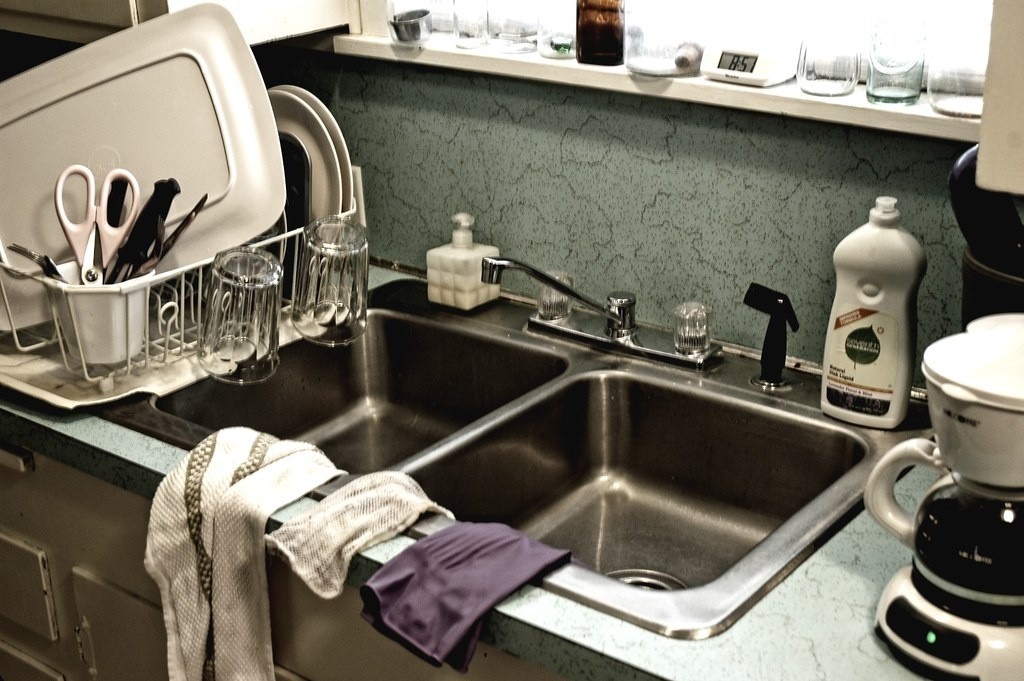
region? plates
[265,82,355,243]
[0,3,286,333]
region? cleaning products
[820,196,928,433]
[426,211,503,310]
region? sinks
[111,309,596,501]
[383,351,938,645]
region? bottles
[819,195,928,429]
[427,213,501,310]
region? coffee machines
[860,313,1024,681]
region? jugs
[864,439,1024,623]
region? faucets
[739,280,802,389]
[479,255,640,350]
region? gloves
[360,520,576,672]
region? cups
[576,0,626,66]
[452,0,490,50]
[794,0,991,119]
[46,258,154,365]
[537,0,576,59]
[389,8,434,43]
[624,0,704,77]
[499,0,538,55]
[290,213,370,347]
[197,246,284,384]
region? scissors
[55,166,139,286]
